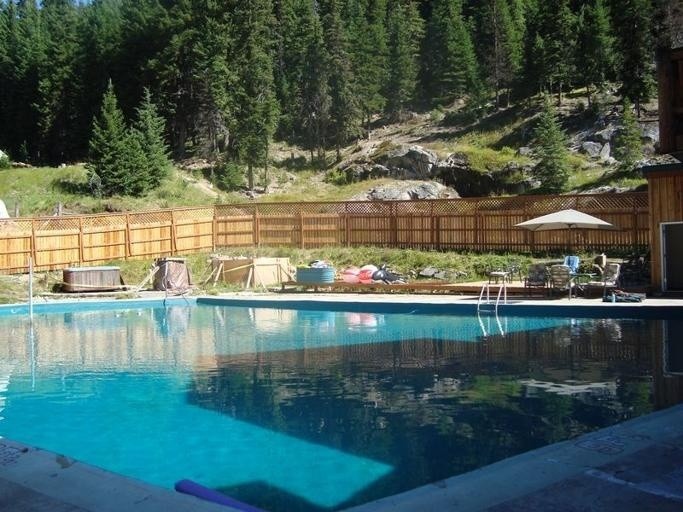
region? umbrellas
[513,206,616,256]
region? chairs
[525,256,621,302]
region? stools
[488,271,510,284]
